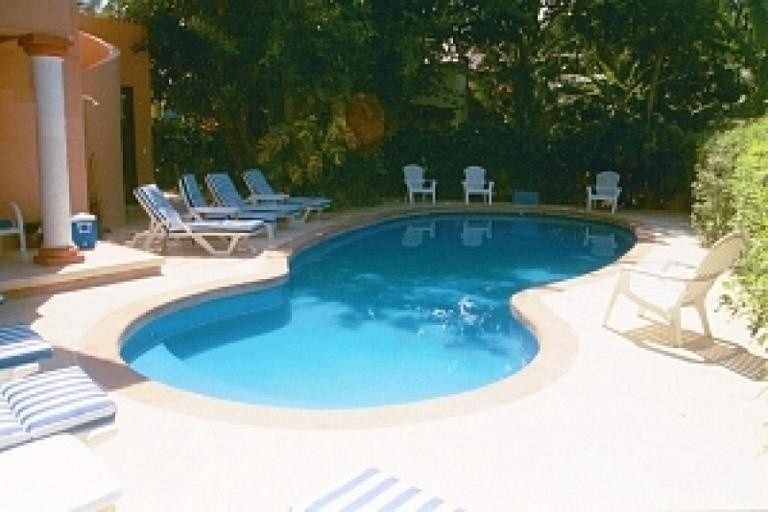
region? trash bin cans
[72,212,98,254]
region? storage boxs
[71,211,98,250]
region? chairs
[461,221,492,248]
[401,163,436,206]
[585,226,618,257]
[461,165,495,205]
[132,167,334,257]
[600,234,745,358]
[401,223,436,247]
[585,171,624,216]
